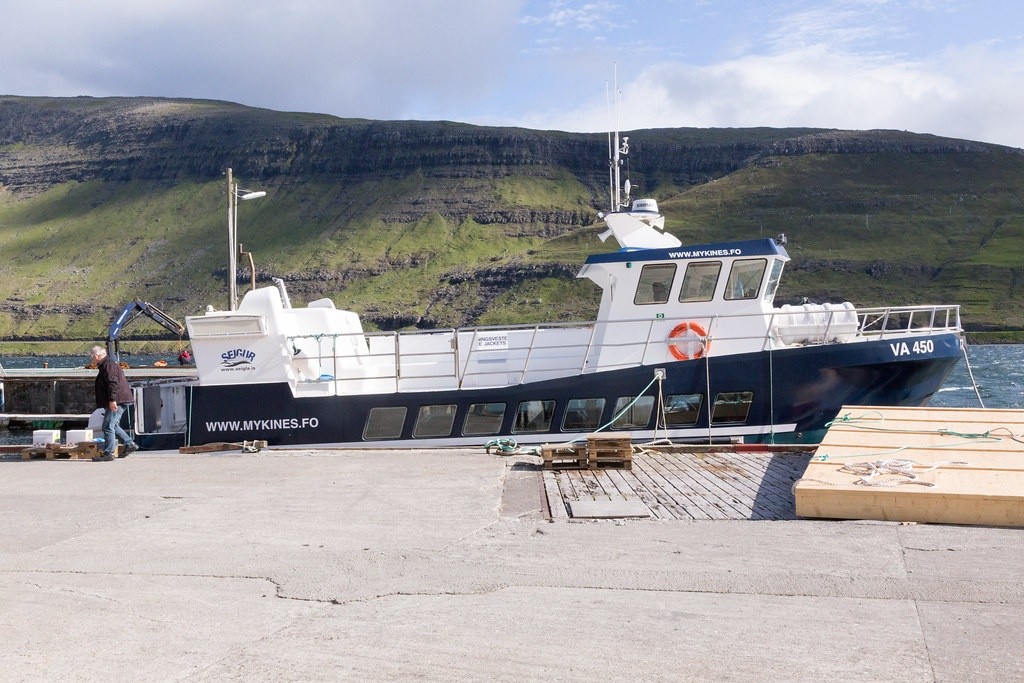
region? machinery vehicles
[107,298,186,364]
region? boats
[133,61,967,446]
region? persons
[886,316,918,330]
[178,350,191,365]
[90,346,138,462]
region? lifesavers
[668,321,711,361]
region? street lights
[227,188,267,310]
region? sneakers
[92,452,114,461]
[118,442,139,458]
